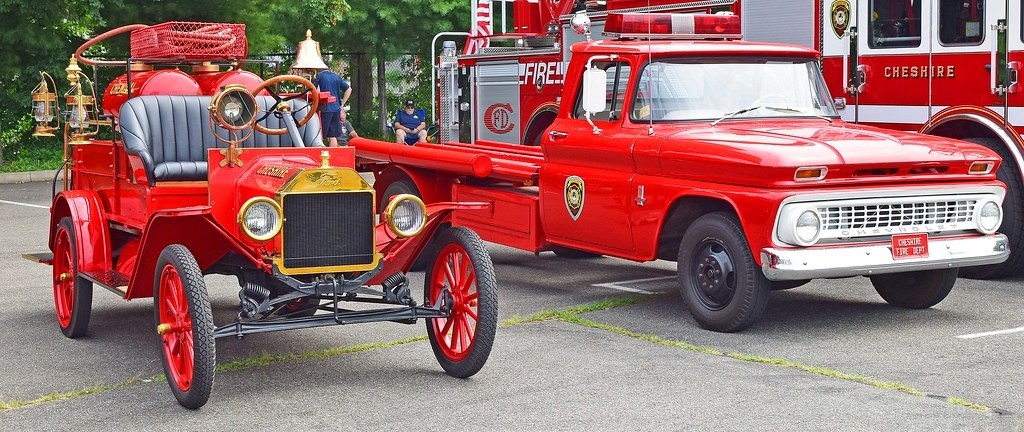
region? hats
[405,100,414,105]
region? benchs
[119,94,326,187]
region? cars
[20,20,499,411]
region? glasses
[407,106,413,109]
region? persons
[313,67,352,146]
[394,100,427,144]
[337,111,359,146]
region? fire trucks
[433,0,1024,280]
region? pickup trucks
[348,41,1010,333]
